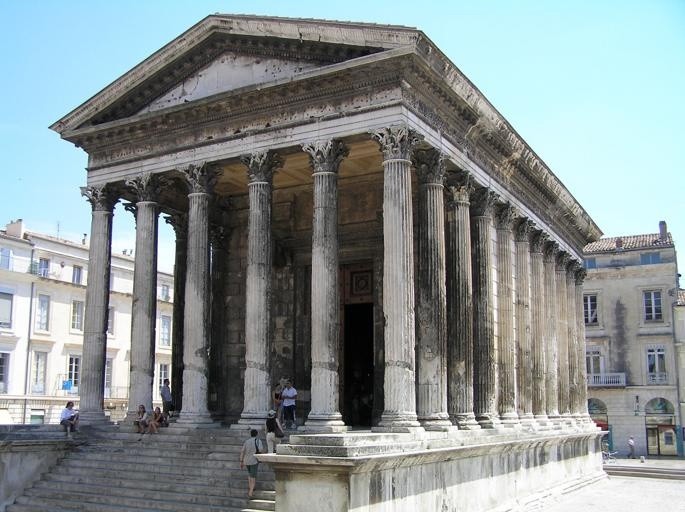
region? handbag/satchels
[275,427,283,437]
[256,452,262,463]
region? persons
[160,379,175,418]
[239,429,264,500]
[264,409,285,453]
[627,435,637,459]
[133,405,164,434]
[60,401,79,437]
[272,381,297,429]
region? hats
[269,410,276,415]
